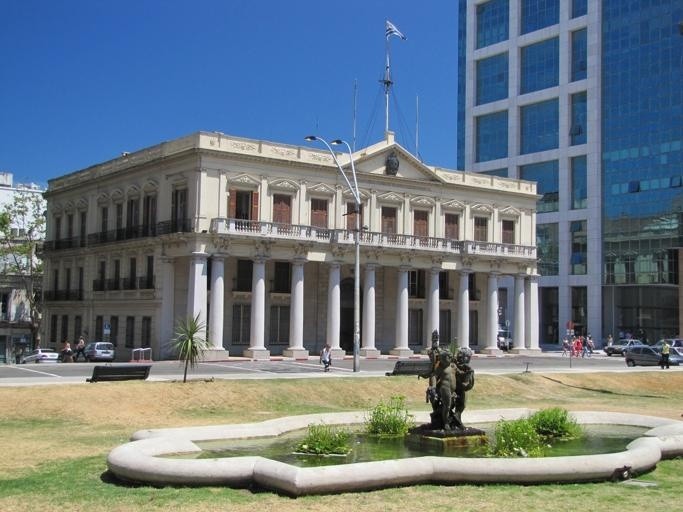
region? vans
[71,342,114,362]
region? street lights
[305,136,361,373]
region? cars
[604,339,683,367]
[21,349,61,363]
[497,329,513,348]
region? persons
[418,347,475,432]
[319,344,332,372]
[74,335,89,362]
[562,327,647,358]
[660,339,670,369]
[60,337,68,351]
[62,340,74,363]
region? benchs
[85,365,152,383]
[384,360,433,377]
